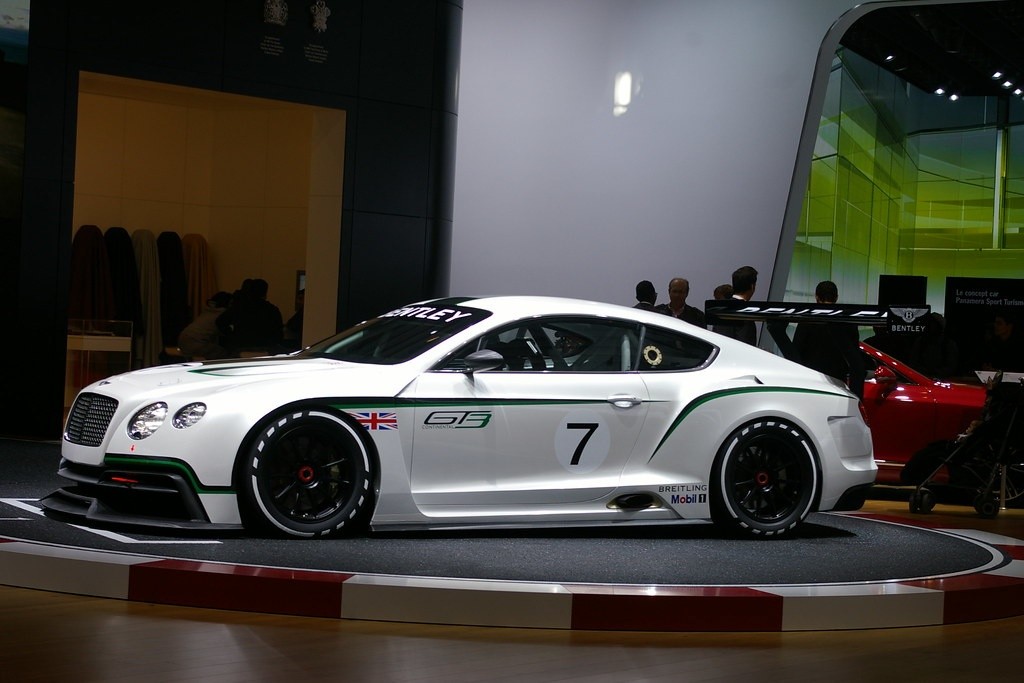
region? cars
[851,340,989,501]
[38,295,878,538]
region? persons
[287,288,303,351]
[792,281,860,384]
[957,420,982,442]
[984,314,1018,372]
[863,313,956,378]
[635,278,707,329]
[178,279,284,360]
[714,267,757,346]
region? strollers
[901,372,1024,518]
